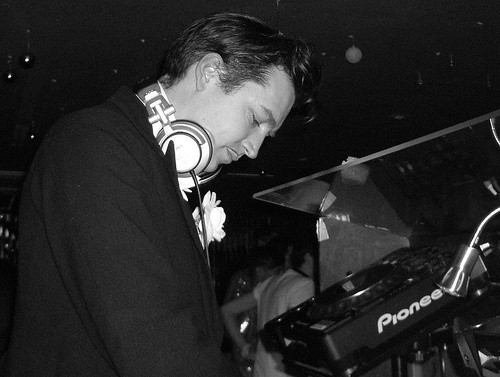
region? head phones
[139,79,213,178]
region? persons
[218,220,326,377]
[4,11,325,377]
[1,173,23,287]
